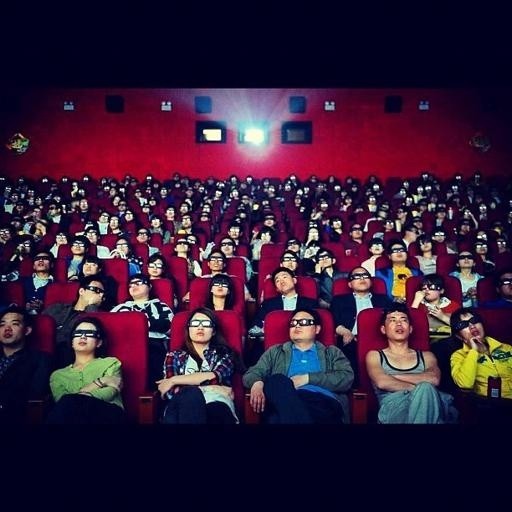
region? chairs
[0,177,512,425]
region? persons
[155,307,242,423]
[242,308,355,423]
[366,307,443,422]
[45,316,125,423]
[449,308,512,422]
[2,303,46,422]
[1,171,511,387]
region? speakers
[289,96,305,112]
[385,95,401,112]
[105,95,124,112]
[195,96,212,113]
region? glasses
[189,320,213,328]
[456,317,479,329]
[0,175,252,294]
[288,319,316,326]
[260,172,512,290]
[72,329,100,339]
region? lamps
[161,101,172,111]
[324,100,335,111]
[63,101,74,110]
[419,100,429,110]
